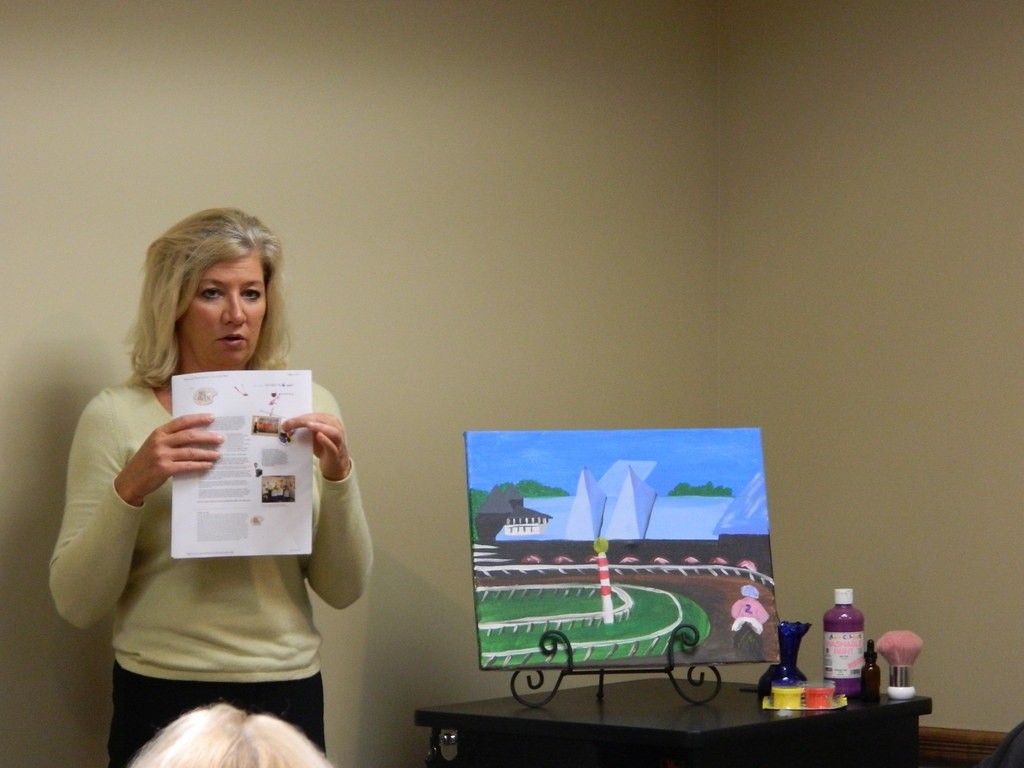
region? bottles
[759,621,812,703]
[824,588,865,698]
[861,639,880,703]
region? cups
[770,685,803,708]
[805,686,835,707]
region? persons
[49,207,374,768]
[125,702,334,768]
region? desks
[413,677,933,767]
